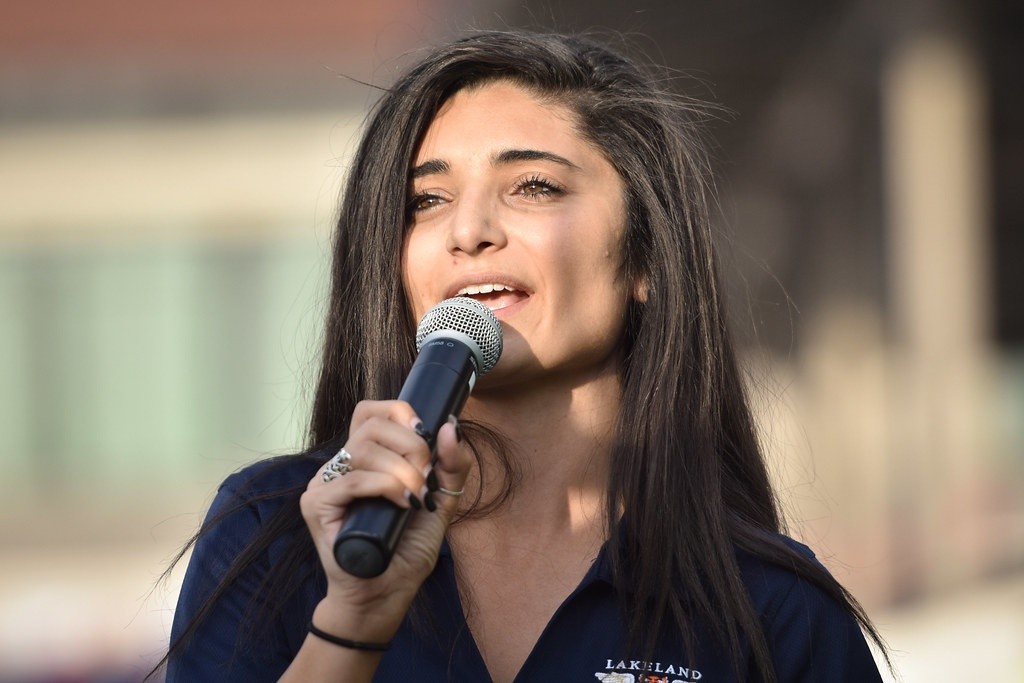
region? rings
[440,488,467,497]
[322,446,354,482]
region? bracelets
[308,620,397,654]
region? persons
[134,28,893,683]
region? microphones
[331,294,502,580]
